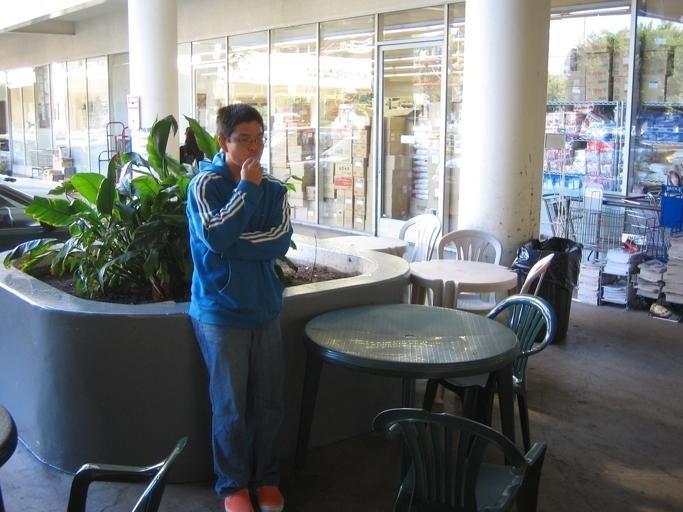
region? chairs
[396,212,443,304]
[437,227,504,318]
[66,438,190,511]
[504,250,556,329]
[372,408,547,511]
[422,293,559,444]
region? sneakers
[255,486,285,512]
[224,481,254,512]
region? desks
[297,302,519,469]
[1,406,19,512]
[409,260,519,330]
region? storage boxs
[545,106,619,195]
[230,94,417,227]
[41,157,76,183]
[560,37,683,100]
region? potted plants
[0,114,411,486]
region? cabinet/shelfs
[541,100,621,245]
[623,102,683,237]
[599,265,638,312]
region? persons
[184,102,296,511]
[661,170,683,200]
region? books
[572,239,682,323]
[624,194,647,201]
[623,198,650,206]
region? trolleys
[539,189,675,265]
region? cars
[0,182,80,254]
[261,126,360,187]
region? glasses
[231,136,266,146]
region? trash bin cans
[517,236,583,343]
